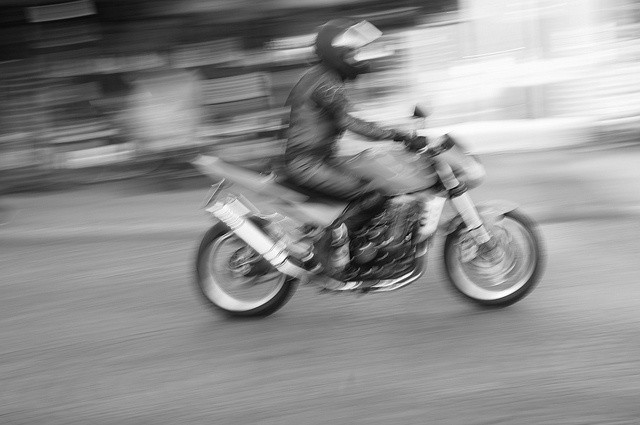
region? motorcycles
[192,106,546,320]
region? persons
[282,16,427,270]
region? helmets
[314,17,385,80]
